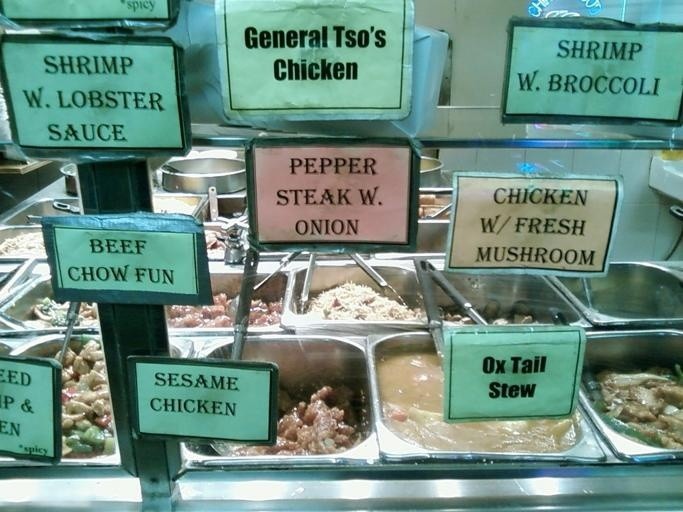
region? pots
[419,155,445,190]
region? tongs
[300,251,409,316]
[0,259,37,295]
